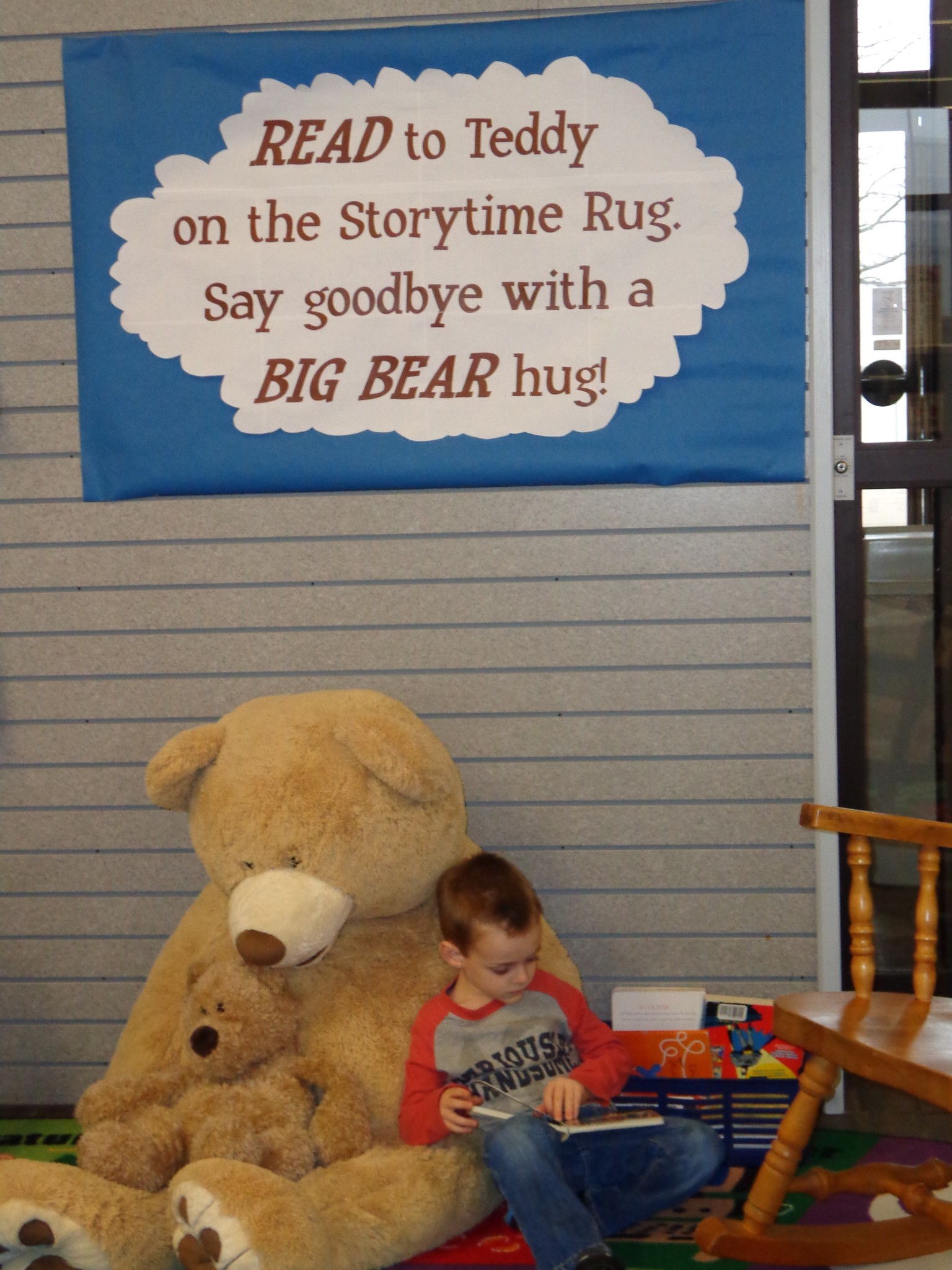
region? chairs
[691,804,952,1268]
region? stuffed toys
[74,952,374,1195]
[0,689,584,1270]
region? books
[610,987,803,1147]
[468,1079,664,1136]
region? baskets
[609,1074,824,1177]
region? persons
[396,853,725,1269]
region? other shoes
[553,1243,625,1270]
[504,1211,518,1229]
